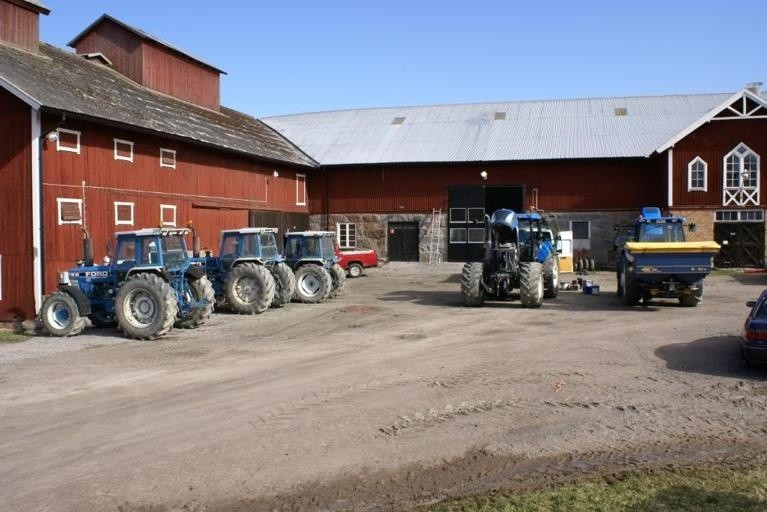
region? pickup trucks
[310,240,378,278]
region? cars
[740,289,767,368]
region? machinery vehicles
[613,207,722,306]
[460,206,562,308]
